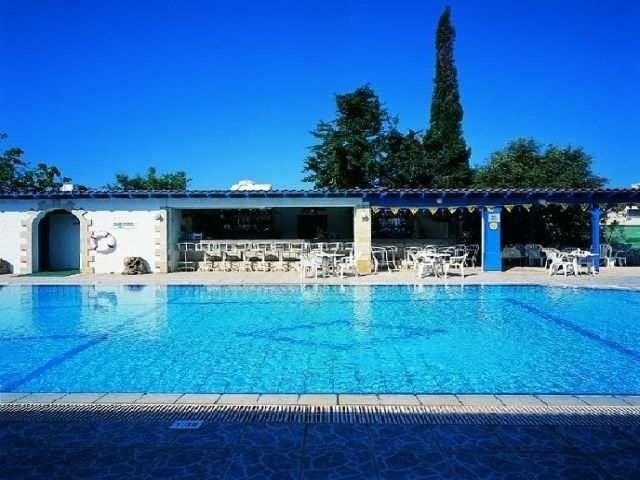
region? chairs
[176,239,632,280]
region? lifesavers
[90,231,116,254]
